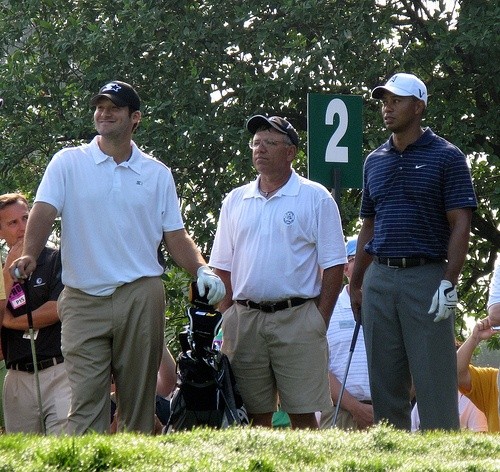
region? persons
[347,73,479,433]
[9,80,227,435]
[0,192,500,437]
[206,114,348,431]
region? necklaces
[259,180,288,197]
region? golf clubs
[332,305,362,429]
[177,331,215,382]
[23,278,47,437]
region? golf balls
[14,268,21,278]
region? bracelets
[27,311,33,329]
